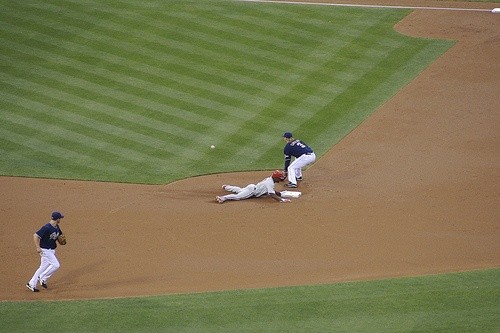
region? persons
[25,212,66,292]
[280,132,317,188]
[215,170,294,204]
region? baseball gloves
[58,235,66,245]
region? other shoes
[222,185,226,190]
[215,196,223,204]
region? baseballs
[210,145,215,148]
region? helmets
[271,170,284,179]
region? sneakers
[25,282,39,292]
[284,182,297,188]
[296,177,303,180]
[38,276,47,288]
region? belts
[305,151,313,155]
[254,186,256,190]
[43,248,55,249]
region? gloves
[280,198,292,203]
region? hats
[282,132,292,138]
[51,212,64,221]
